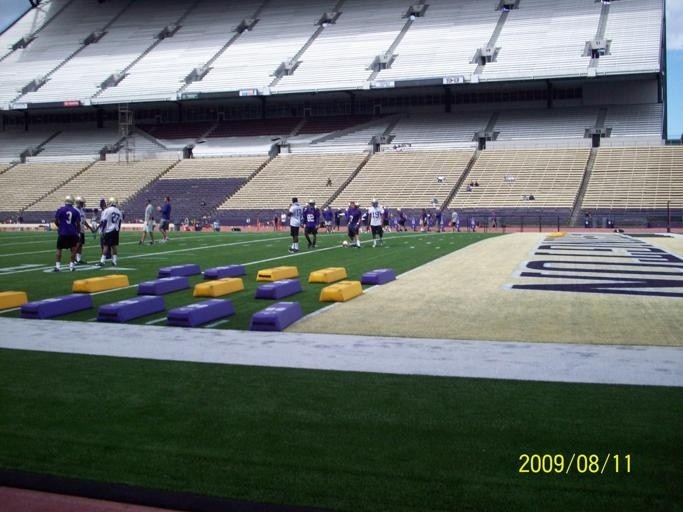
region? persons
[366,198,386,248]
[50,191,220,273]
[3,213,24,225]
[344,201,362,248]
[243,176,537,232]
[287,198,301,254]
[300,199,320,248]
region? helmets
[370,198,378,204]
[107,196,118,207]
[62,194,74,204]
[74,196,86,208]
[307,198,316,206]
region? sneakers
[288,239,384,256]
[50,235,166,274]
